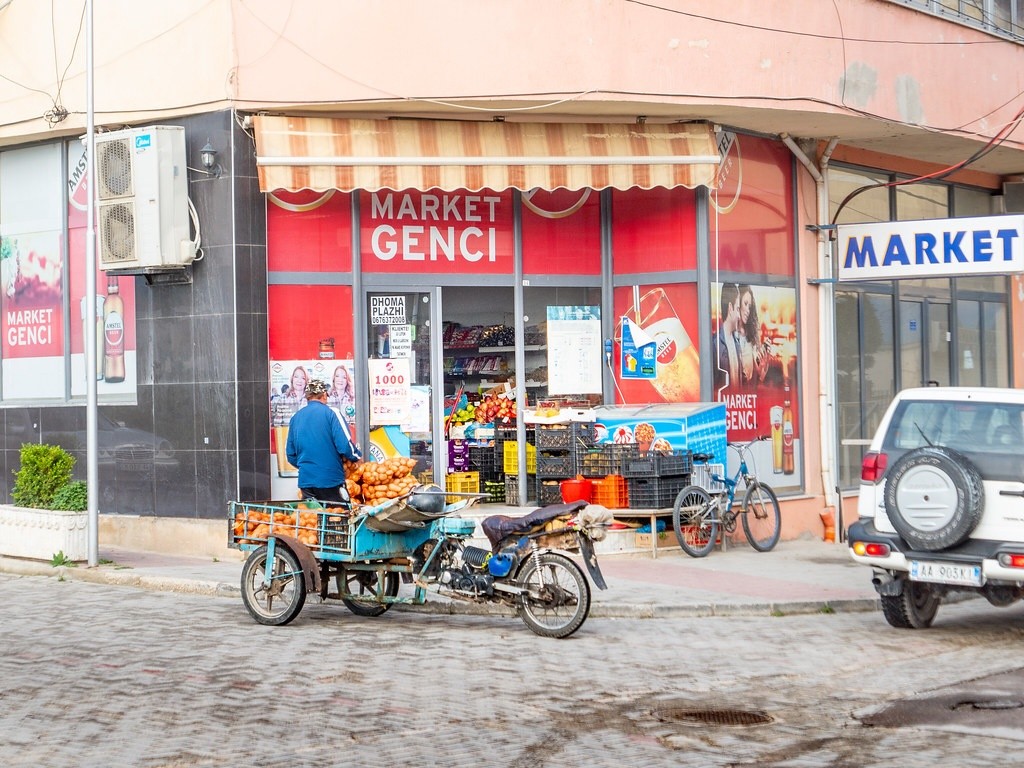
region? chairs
[946,429,989,450]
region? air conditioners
[93,125,193,272]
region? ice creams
[613,422,674,458]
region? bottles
[319,338,335,360]
[756,338,771,366]
[103,275,125,383]
[782,387,794,475]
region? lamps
[197,137,216,178]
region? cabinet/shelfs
[477,344,547,389]
[418,341,503,381]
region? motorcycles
[225,486,612,639]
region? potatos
[234,508,349,548]
[298,455,420,510]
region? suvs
[846,381,1024,630]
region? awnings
[252,116,721,192]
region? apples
[446,404,476,422]
[475,396,517,424]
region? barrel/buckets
[559,479,591,504]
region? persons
[280,366,308,401]
[270,388,280,401]
[286,380,362,511]
[713,283,781,388]
[327,366,355,406]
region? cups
[274,424,299,478]
[81,294,106,381]
[614,288,700,402]
[770,406,784,473]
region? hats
[305,380,330,400]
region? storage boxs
[444,395,468,416]
[481,382,526,399]
[418,469,480,504]
[446,455,469,472]
[578,528,637,554]
[469,426,725,509]
[635,531,683,547]
[681,525,721,546]
[445,438,469,454]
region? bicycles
[672,435,782,558]
